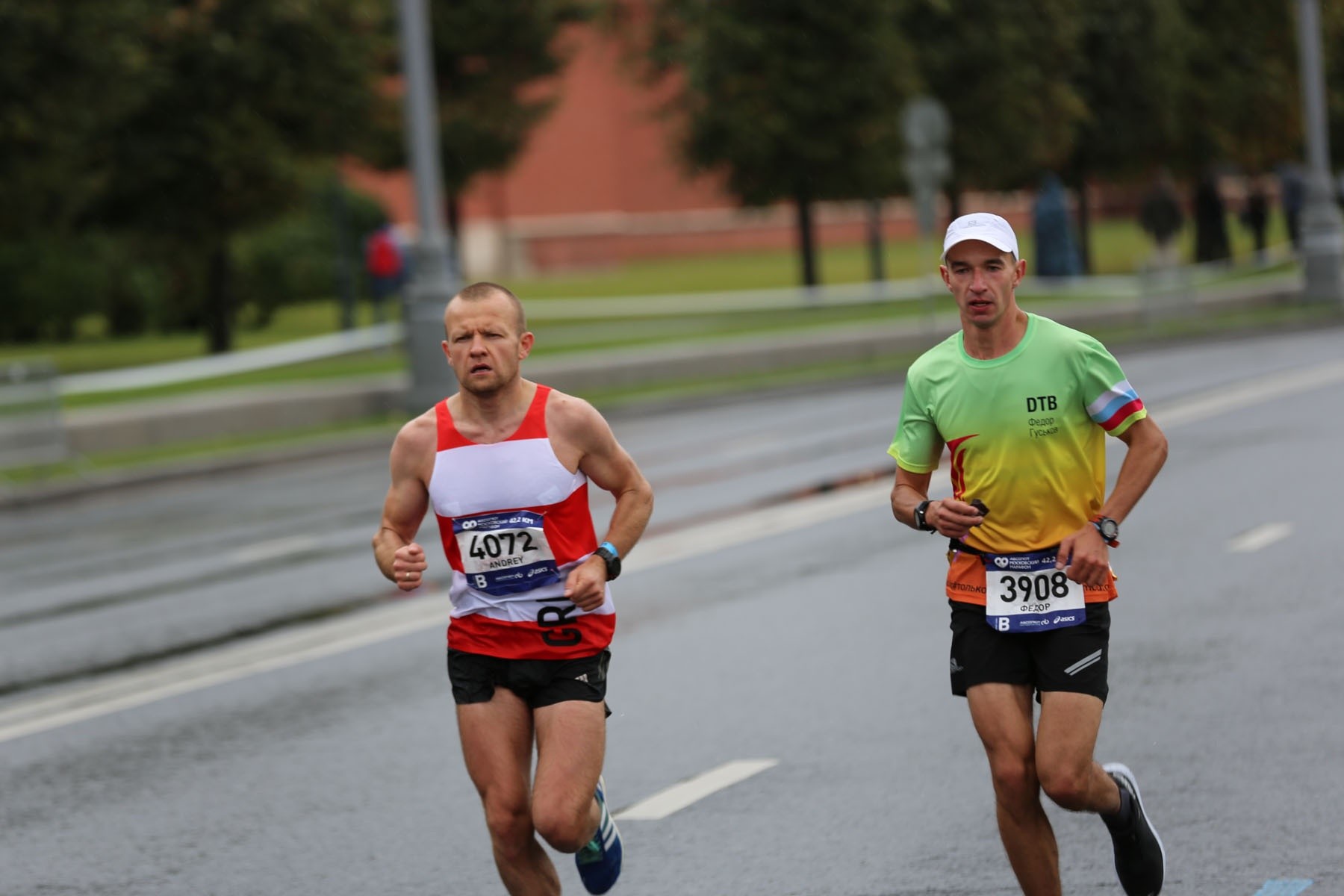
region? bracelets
[600,541,619,557]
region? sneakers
[1097,763,1166,896]
[575,774,624,896]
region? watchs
[1088,513,1120,548]
[593,547,622,582]
[913,499,938,535]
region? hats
[938,210,1020,264]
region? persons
[372,283,656,895]
[1137,174,1304,274]
[885,213,1173,896]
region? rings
[406,571,411,581]
